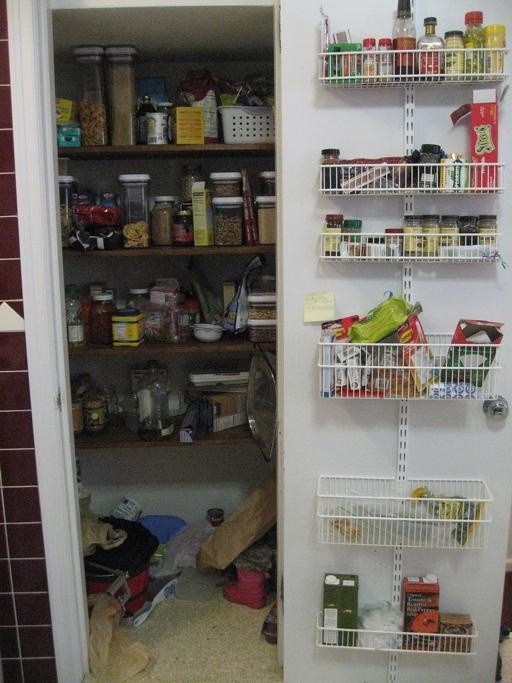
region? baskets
[317,332,503,402]
[316,474,494,551]
[319,163,505,196]
[318,38,508,90]
[218,103,275,145]
[315,609,478,656]
[318,232,500,265]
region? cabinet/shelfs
[303,35,508,658]
[52,134,276,455]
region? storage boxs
[185,387,249,436]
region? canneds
[325,214,496,260]
[320,149,340,164]
[88,295,115,348]
[156,102,174,113]
[82,398,107,436]
[127,287,150,310]
[174,210,194,246]
[146,112,169,145]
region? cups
[72,44,138,146]
[117,173,151,249]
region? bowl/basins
[191,323,226,341]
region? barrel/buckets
[85,513,152,619]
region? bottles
[57,173,75,247]
[320,147,340,193]
[324,213,496,261]
[376,38,392,83]
[389,144,440,189]
[100,383,120,432]
[65,284,87,349]
[394,0,504,81]
[155,101,173,143]
[206,507,224,527]
[182,161,276,246]
[361,37,375,86]
[137,95,156,139]
